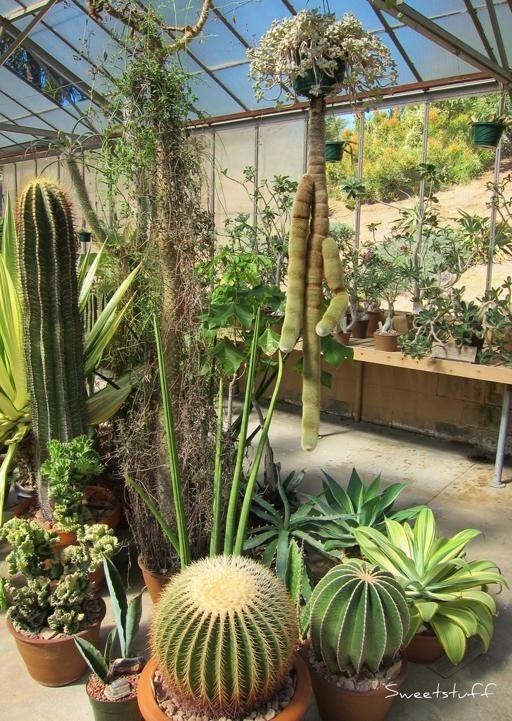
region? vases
[292,59,346,99]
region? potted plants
[325,139,358,165]
[468,114,511,148]
[0,175,512,720]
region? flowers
[246,7,399,112]
